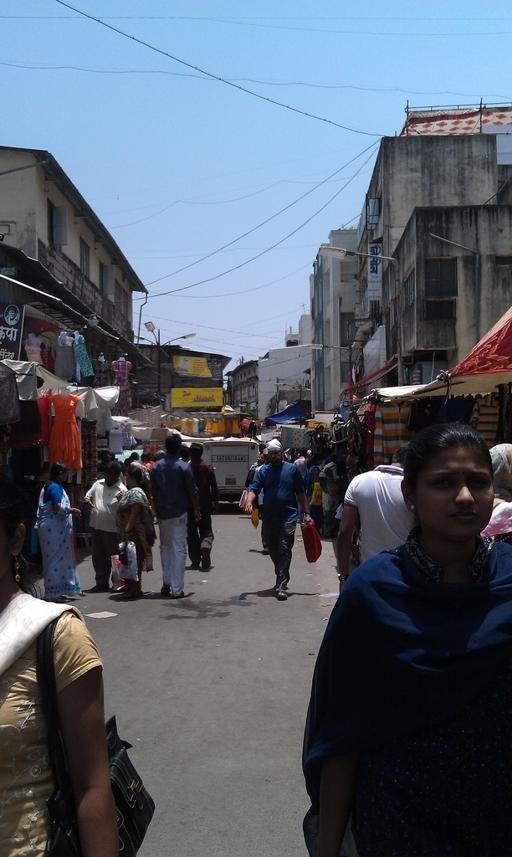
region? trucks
[202,436,261,508]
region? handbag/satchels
[36,716,155,857]
[300,515,322,562]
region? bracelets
[337,574,347,583]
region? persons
[334,441,418,599]
[82,426,220,603]
[297,419,510,856]
[0,458,125,856]
[33,460,87,604]
[474,441,511,553]
[243,437,314,600]
[46,386,83,467]
[239,435,344,541]
[5,305,19,325]
[23,328,134,407]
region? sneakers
[161,586,184,598]
[88,585,143,601]
[277,591,287,600]
[192,547,211,568]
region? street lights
[273,382,288,414]
[317,244,404,389]
[305,342,353,404]
[133,320,199,403]
